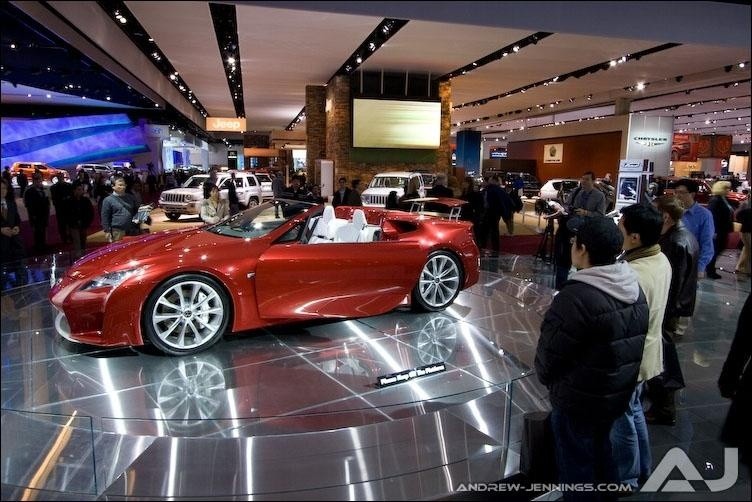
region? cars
[420,168,618,205]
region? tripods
[535,218,554,263]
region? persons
[0,159,751,502]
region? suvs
[671,139,691,159]
[359,170,426,209]
[8,160,70,186]
[154,169,265,224]
[656,175,749,211]
[75,162,284,198]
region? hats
[567,215,623,257]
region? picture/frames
[542,142,564,164]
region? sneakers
[644,412,676,426]
[707,273,722,280]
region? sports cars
[43,301,476,441]
[46,194,485,358]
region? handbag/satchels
[129,204,153,227]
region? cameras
[569,207,577,212]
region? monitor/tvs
[691,171,704,178]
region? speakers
[519,412,552,472]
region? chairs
[309,206,348,244]
[334,209,383,244]
